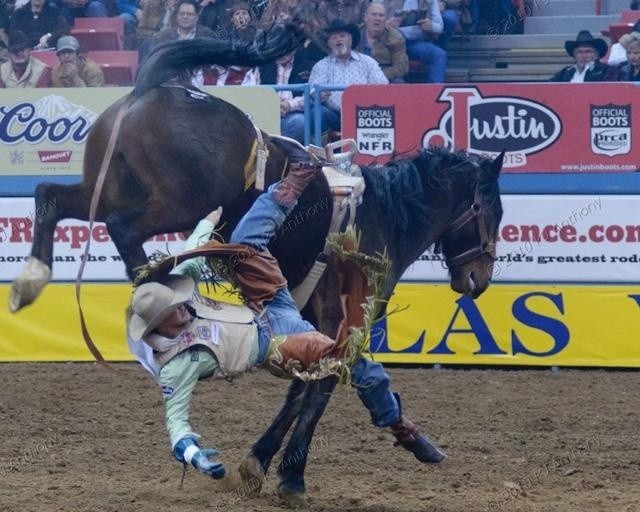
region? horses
[7,21,508,508]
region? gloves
[172,434,227,480]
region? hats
[122,274,194,343]
[55,35,81,55]
[7,31,32,51]
[321,19,361,49]
[564,30,609,59]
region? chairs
[598,9,640,81]
[0,5,423,85]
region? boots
[390,423,446,465]
[269,134,320,205]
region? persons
[126,133,445,478]
[543,1,640,82]
[2,0,535,145]
